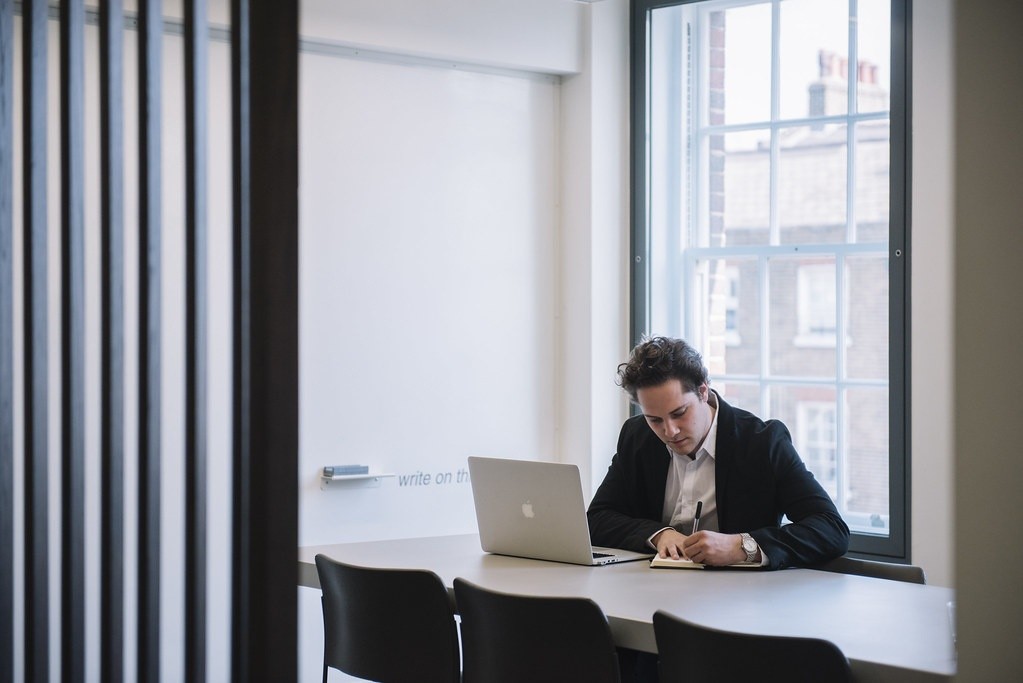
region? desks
[298,533,956,683]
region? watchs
[740,533,757,563]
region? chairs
[315,554,460,683]
[453,577,622,683]
[653,610,854,683]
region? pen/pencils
[691,500,704,537]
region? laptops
[467,456,656,567]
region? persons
[587,338,852,569]
